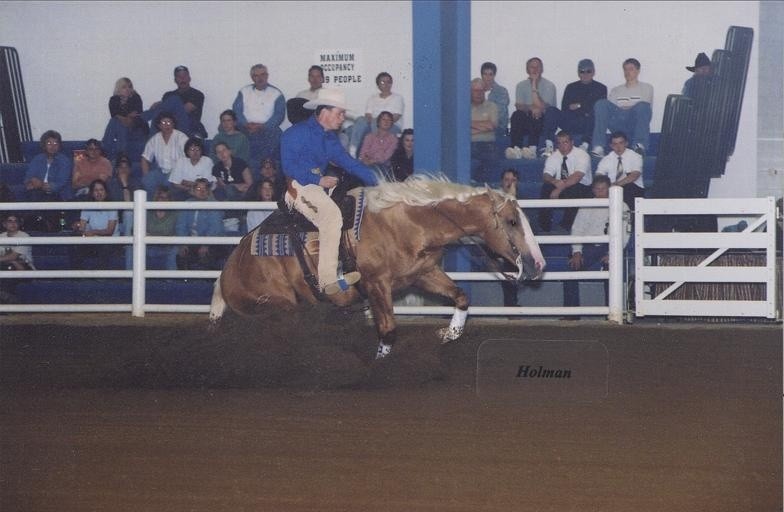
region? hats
[175,65,189,72]
[301,87,352,111]
[578,59,594,70]
[685,52,712,72]
[116,154,132,168]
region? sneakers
[504,147,521,161]
[541,147,554,158]
[590,146,604,159]
[522,147,537,161]
[324,269,363,297]
[634,143,646,158]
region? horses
[209,165,548,361]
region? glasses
[604,222,610,234]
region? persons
[686,53,712,78]
[1,56,415,297]
[470,56,652,306]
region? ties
[561,155,569,179]
[616,156,624,183]
